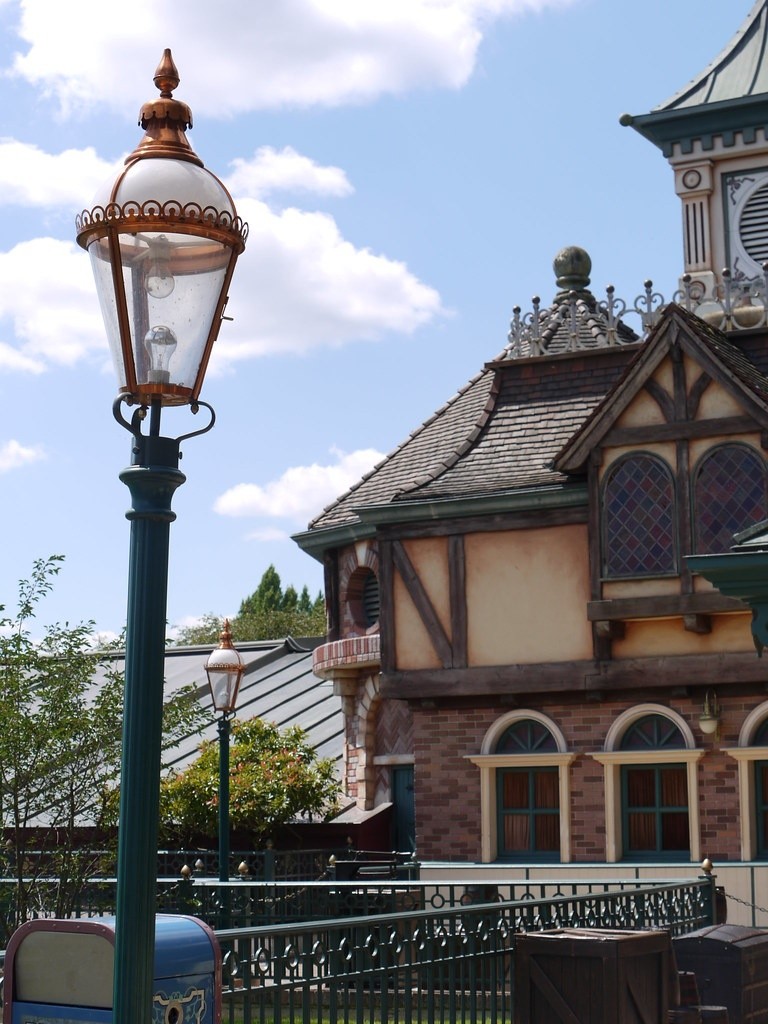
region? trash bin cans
[1,912,223,1024]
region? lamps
[698,686,721,746]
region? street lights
[77,46,249,1024]
[205,618,249,982]
